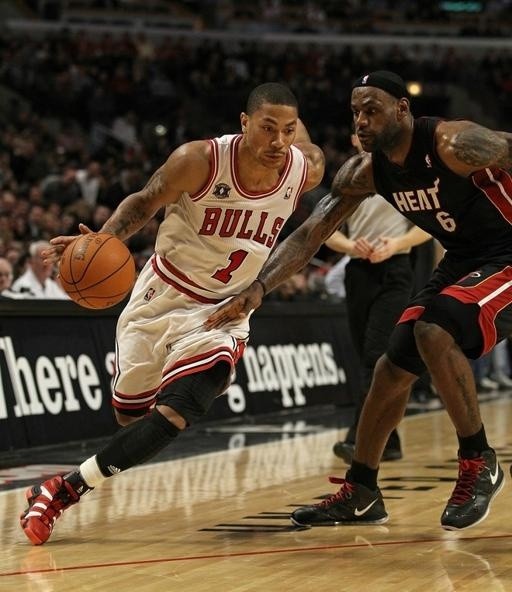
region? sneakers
[21,474,79,545]
[440,448,505,531]
[291,476,389,527]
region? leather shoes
[333,439,355,458]
[382,448,403,461]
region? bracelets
[252,278,267,298]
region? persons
[0,257,14,301]
[324,123,433,467]
[1,23,205,82]
[89,139,118,227]
[19,84,326,546]
[0,82,88,238]
[204,70,512,532]
[5,239,23,265]
[89,82,204,139]
[12,240,71,301]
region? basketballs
[60,232,136,310]
